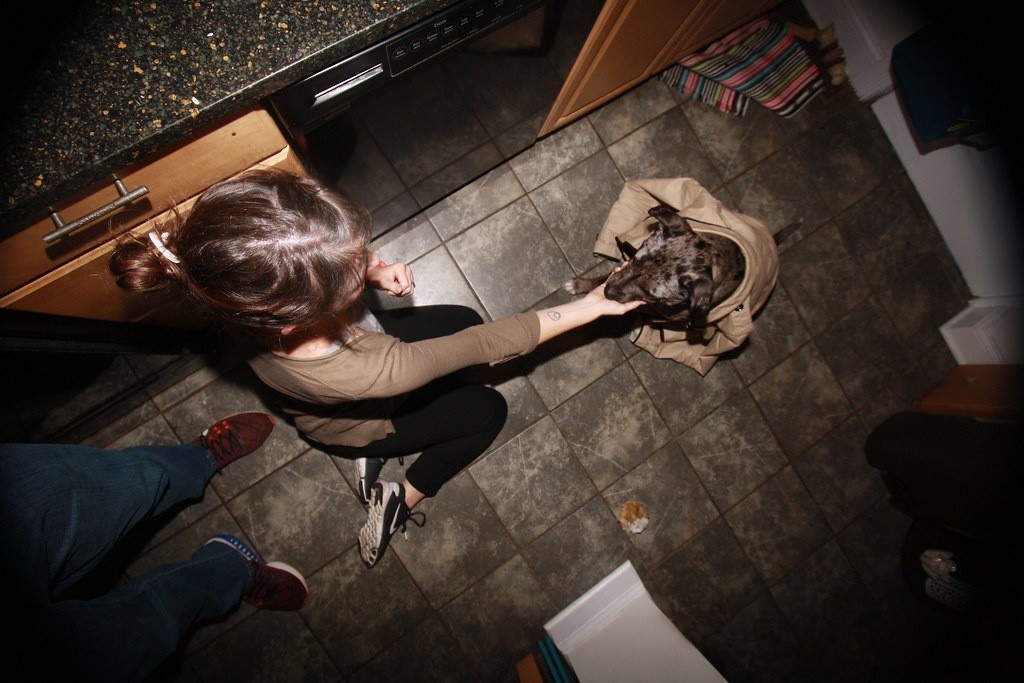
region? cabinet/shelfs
[0,97,309,335]
[536,0,785,139]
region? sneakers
[357,481,426,570]
[353,456,386,505]
[192,412,274,474]
[204,538,307,613]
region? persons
[106,169,651,566]
[0,410,309,683]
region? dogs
[563,203,801,331]
